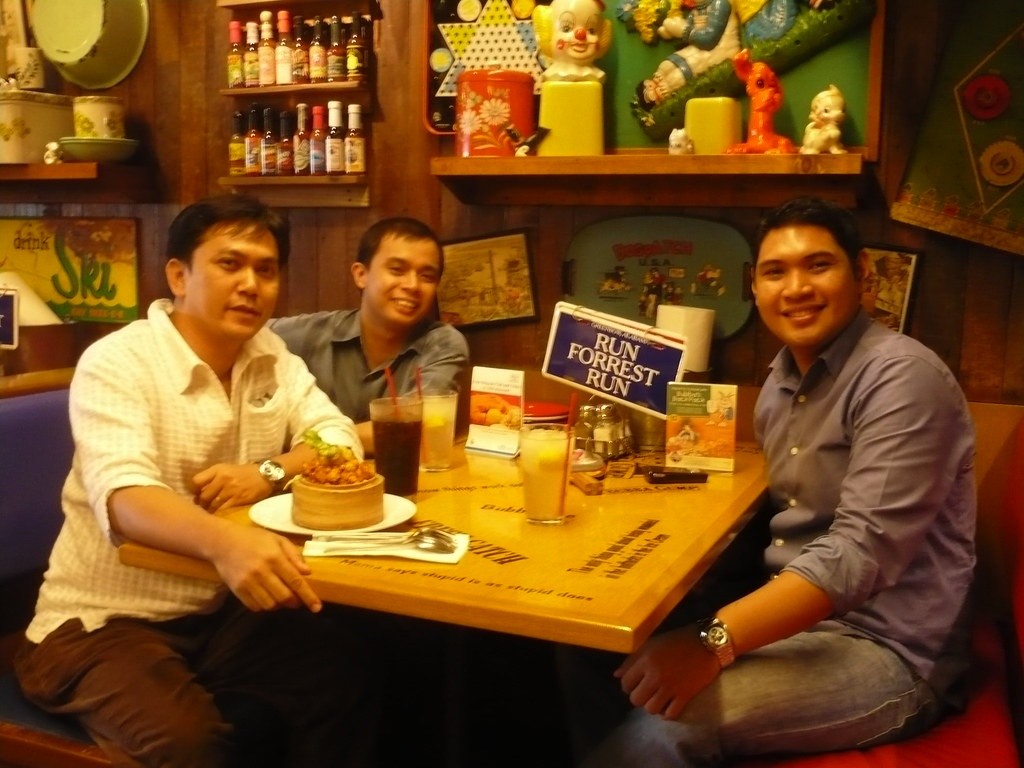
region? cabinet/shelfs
[215,0,385,208]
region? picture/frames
[854,240,924,336]
[433,227,540,331]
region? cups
[370,396,423,503]
[413,391,458,472]
[519,424,576,525]
[72,97,125,141]
[16,48,46,90]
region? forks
[327,529,451,544]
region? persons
[557,194,979,768]
[272,216,470,458]
[13,194,383,768]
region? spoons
[325,538,453,554]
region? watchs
[697,616,736,668]
[255,457,286,495]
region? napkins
[302,532,470,566]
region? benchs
[0,367,129,768]
[742,417,1024,768]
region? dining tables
[120,423,772,768]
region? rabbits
[728,48,795,153]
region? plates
[247,493,416,537]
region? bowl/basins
[58,137,138,163]
[32,0,150,90]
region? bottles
[228,101,367,176]
[575,405,632,456]
[227,11,367,89]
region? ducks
[797,84,846,155]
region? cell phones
[647,467,709,484]
